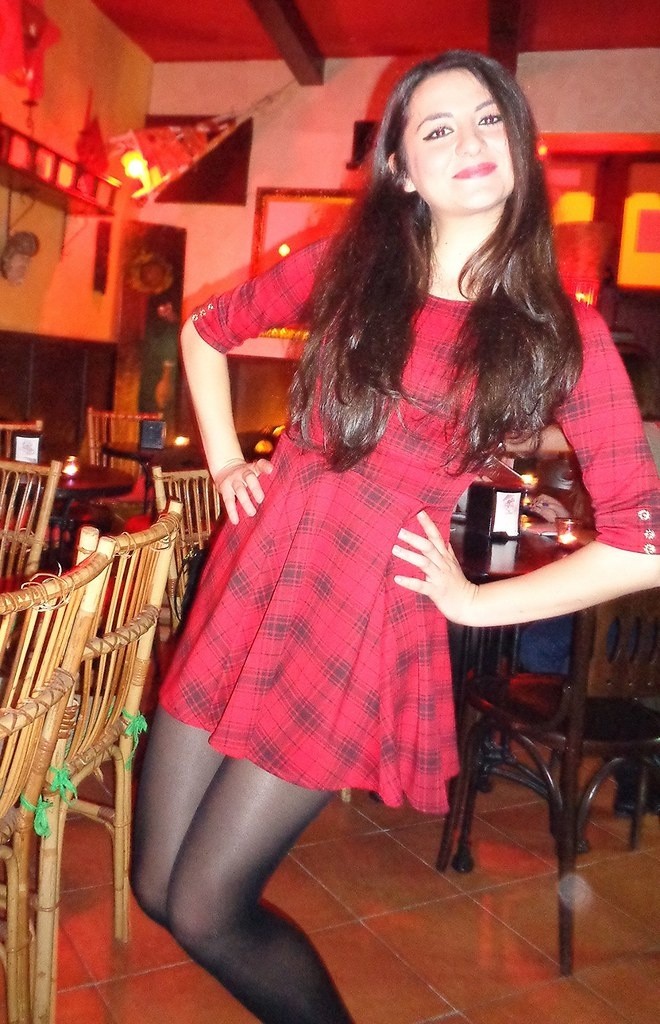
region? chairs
[0,405,222,1024]
[454,585,660,974]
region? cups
[556,516,583,550]
[60,455,79,479]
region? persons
[516,353,660,814]
[134,258,180,413]
[129,49,660,1024]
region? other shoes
[614,787,660,816]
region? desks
[443,514,581,796]
[102,440,157,471]
[147,445,207,519]
[12,461,134,566]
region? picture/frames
[248,187,356,341]
